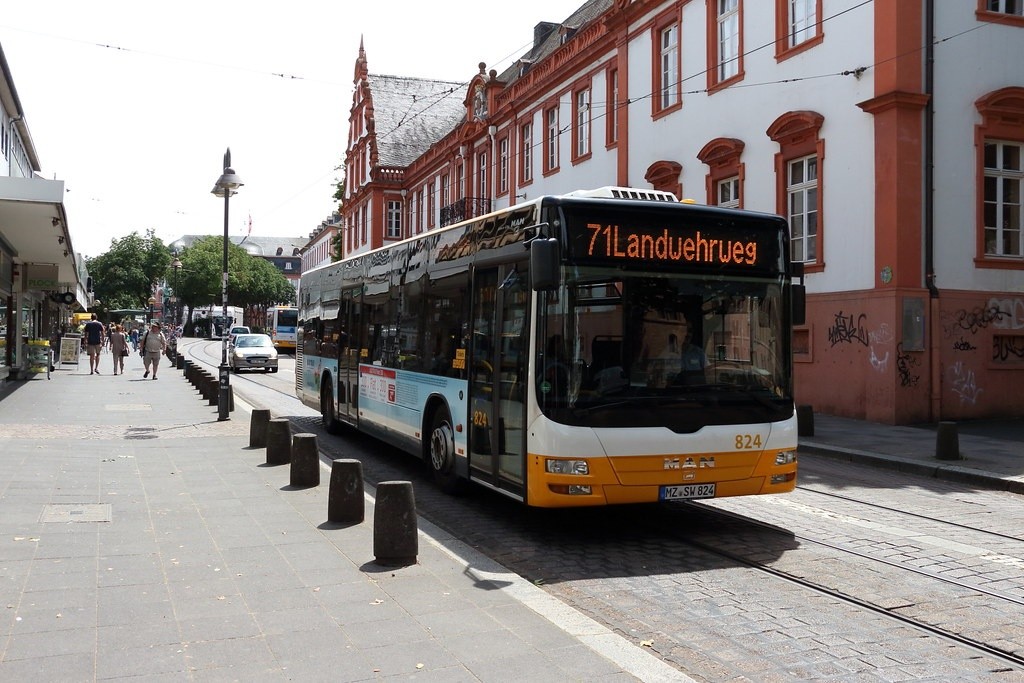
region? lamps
[57,236,67,245]
[63,250,71,258]
[52,216,62,227]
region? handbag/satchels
[122,350,128,356]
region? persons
[109,325,129,375]
[67,318,184,354]
[260,327,271,337]
[84,313,105,375]
[303,331,338,358]
[655,319,710,380]
[245,339,249,345]
[192,324,207,338]
[140,322,166,380]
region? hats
[151,322,159,327]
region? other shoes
[95,369,100,374]
[90,372,93,375]
[153,375,158,380]
[143,371,150,378]
[114,374,117,375]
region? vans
[227,325,252,349]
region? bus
[270,306,299,352]
[293,184,808,510]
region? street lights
[210,146,245,421]
[172,248,183,337]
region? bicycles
[104,335,142,352]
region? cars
[228,334,279,374]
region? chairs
[324,324,571,394]
